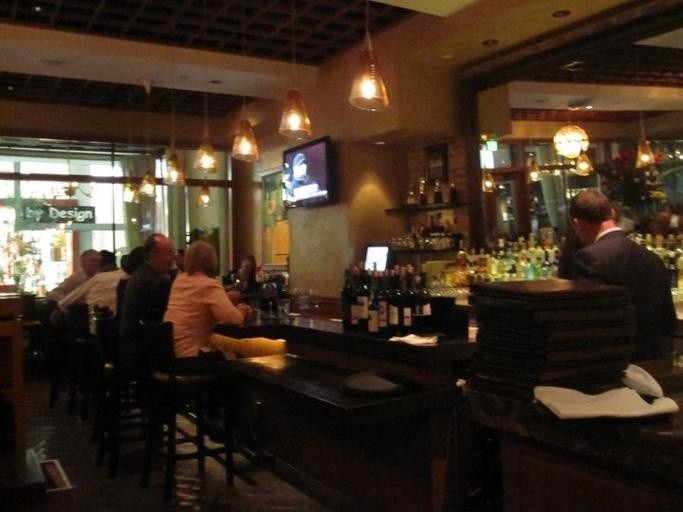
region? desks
[236,352,462,511]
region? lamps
[230,1,258,166]
[346,0,391,115]
[119,78,220,210]
[551,106,590,157]
[279,0,312,146]
[481,155,497,193]
[633,109,655,168]
[574,111,594,176]
[526,115,543,184]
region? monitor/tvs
[280,134,332,208]
[362,245,396,274]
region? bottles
[416,178,426,205]
[340,267,434,336]
[389,232,455,251]
[433,177,441,203]
[405,182,416,206]
[627,233,682,288]
[440,230,560,287]
[447,183,457,203]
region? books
[467,278,640,401]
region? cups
[277,299,290,319]
[293,287,312,309]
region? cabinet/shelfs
[383,199,467,255]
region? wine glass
[260,282,277,316]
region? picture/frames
[257,167,291,271]
[421,141,449,183]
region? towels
[531,381,681,426]
[388,330,440,348]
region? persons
[161,242,253,442]
[628,199,683,236]
[186,227,207,244]
[44,245,150,341]
[566,190,677,361]
[287,151,321,197]
[122,232,175,380]
[226,254,257,290]
